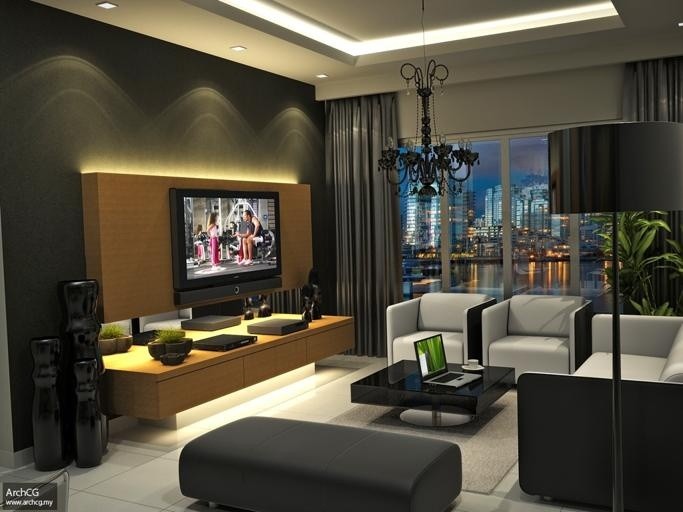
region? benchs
[177,415,462,512]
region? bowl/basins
[159,352,187,365]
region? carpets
[327,392,517,496]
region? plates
[462,365,484,371]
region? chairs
[386,293,592,385]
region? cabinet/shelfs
[99,311,356,420]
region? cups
[468,359,478,368]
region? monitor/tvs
[168,187,284,308]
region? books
[192,334,259,352]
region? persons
[229,209,265,267]
[194,223,207,263]
[206,211,222,267]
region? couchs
[515,313,682,510]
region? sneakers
[244,260,254,265]
[211,266,224,272]
[238,260,248,265]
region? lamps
[374,2,480,200]
[546,120,682,509]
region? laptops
[412,334,483,390]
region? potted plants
[146,321,193,359]
[96,324,132,355]
